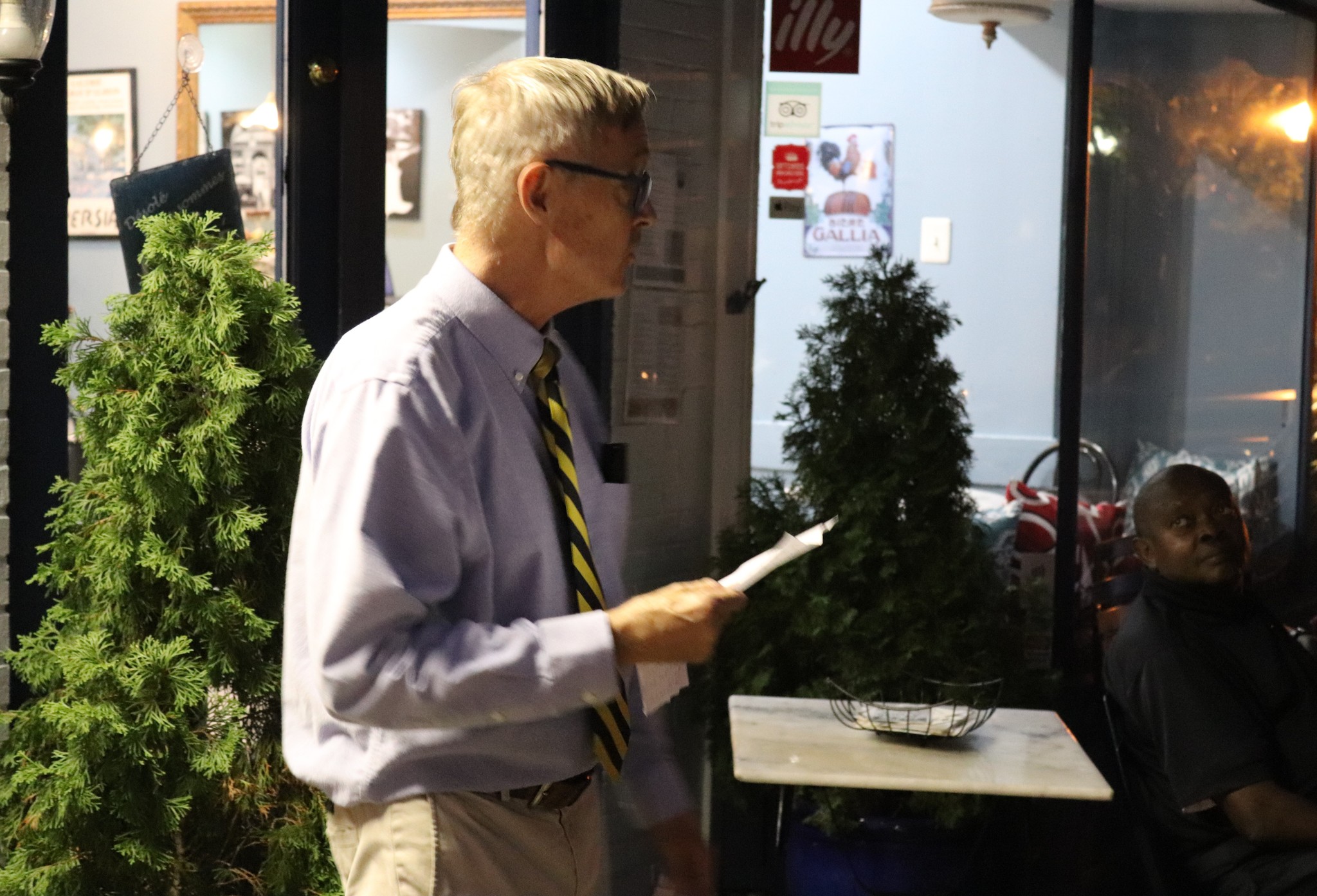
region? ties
[533,337,634,783]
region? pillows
[1007,434,1277,568]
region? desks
[723,689,1116,896]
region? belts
[481,768,598,810]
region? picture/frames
[67,68,136,239]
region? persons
[1100,465,1317,896]
[282,55,748,896]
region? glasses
[539,158,652,212]
